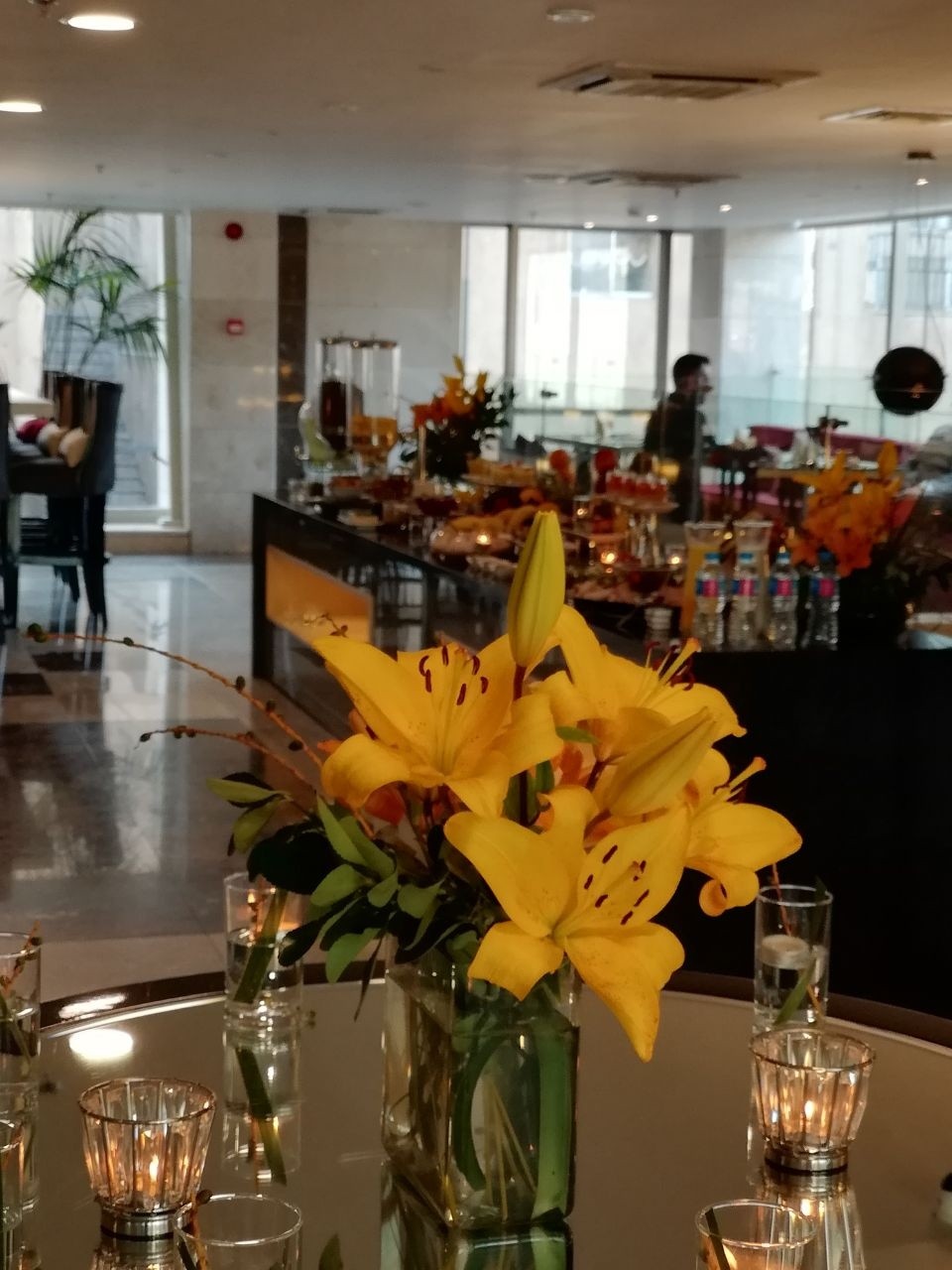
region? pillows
[59,427,90,467]
[37,421,69,457]
[16,418,48,441]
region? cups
[223,872,305,1027]
[0,932,45,1100]
[0,1099,41,1212]
[752,886,833,1032]
[76,1075,217,1241]
[752,1165,866,1270]
[749,1029,878,1177]
[693,1198,817,1270]
[90,1226,208,1270]
[220,1017,304,1181]
[174,1193,303,1270]
[0,1112,31,1232]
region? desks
[534,425,922,529]
[34,980,952,1269]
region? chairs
[0,371,123,629]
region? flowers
[784,442,919,576]
[29,510,802,1224]
[548,445,652,533]
[400,355,519,486]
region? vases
[383,961,582,1241]
[838,573,911,650]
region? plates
[336,516,387,530]
[466,554,517,576]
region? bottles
[767,551,800,647]
[692,554,726,646]
[807,551,838,646]
[734,518,774,636]
[680,519,725,634]
[728,553,760,647]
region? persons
[642,351,757,524]
